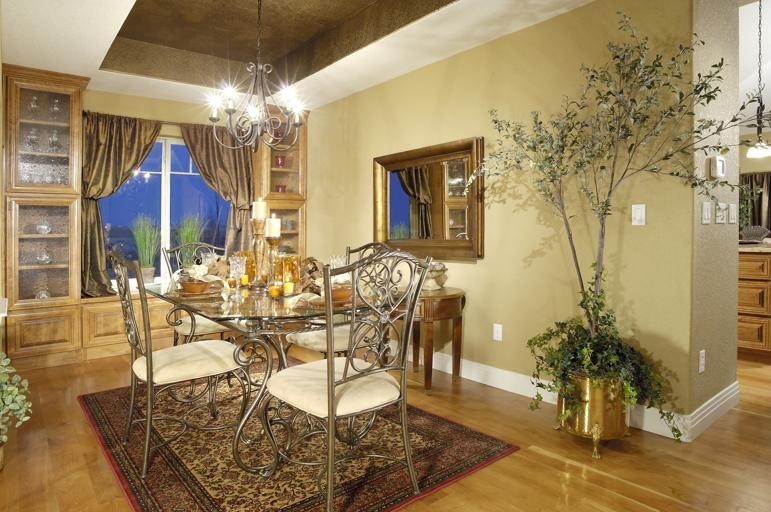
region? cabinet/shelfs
[426,158,469,240]
[737,253,771,358]
[261,200,309,264]
[1,193,81,310]
[257,103,310,200]
[83,296,178,361]
[1,61,90,193]
[6,304,83,372]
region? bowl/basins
[331,284,353,300]
[179,280,210,293]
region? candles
[253,198,269,222]
[267,214,282,238]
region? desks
[381,279,464,389]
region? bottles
[48,95,65,122]
[25,124,42,152]
[48,127,64,154]
[27,94,43,121]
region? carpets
[74,356,520,511]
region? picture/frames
[374,137,484,263]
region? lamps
[204,0,307,153]
[747,1,770,160]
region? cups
[275,184,286,192]
[275,156,286,167]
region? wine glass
[229,255,247,303]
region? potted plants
[176,212,210,273]
[130,212,159,283]
[1,351,37,473]
[466,9,771,459]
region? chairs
[740,224,771,242]
